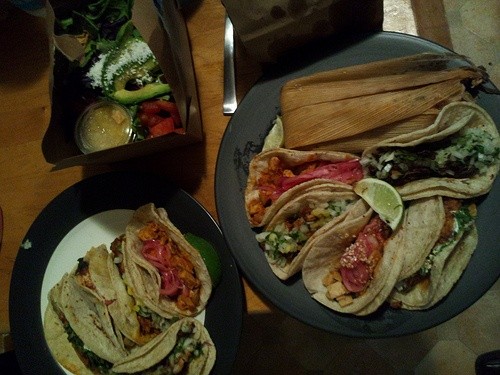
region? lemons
[353,177,405,231]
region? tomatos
[140,100,185,138]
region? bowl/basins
[75,101,137,156]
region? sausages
[271,160,363,200]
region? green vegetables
[371,132,500,182]
[58,0,145,67]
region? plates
[8,168,245,375]
[215,30,500,338]
